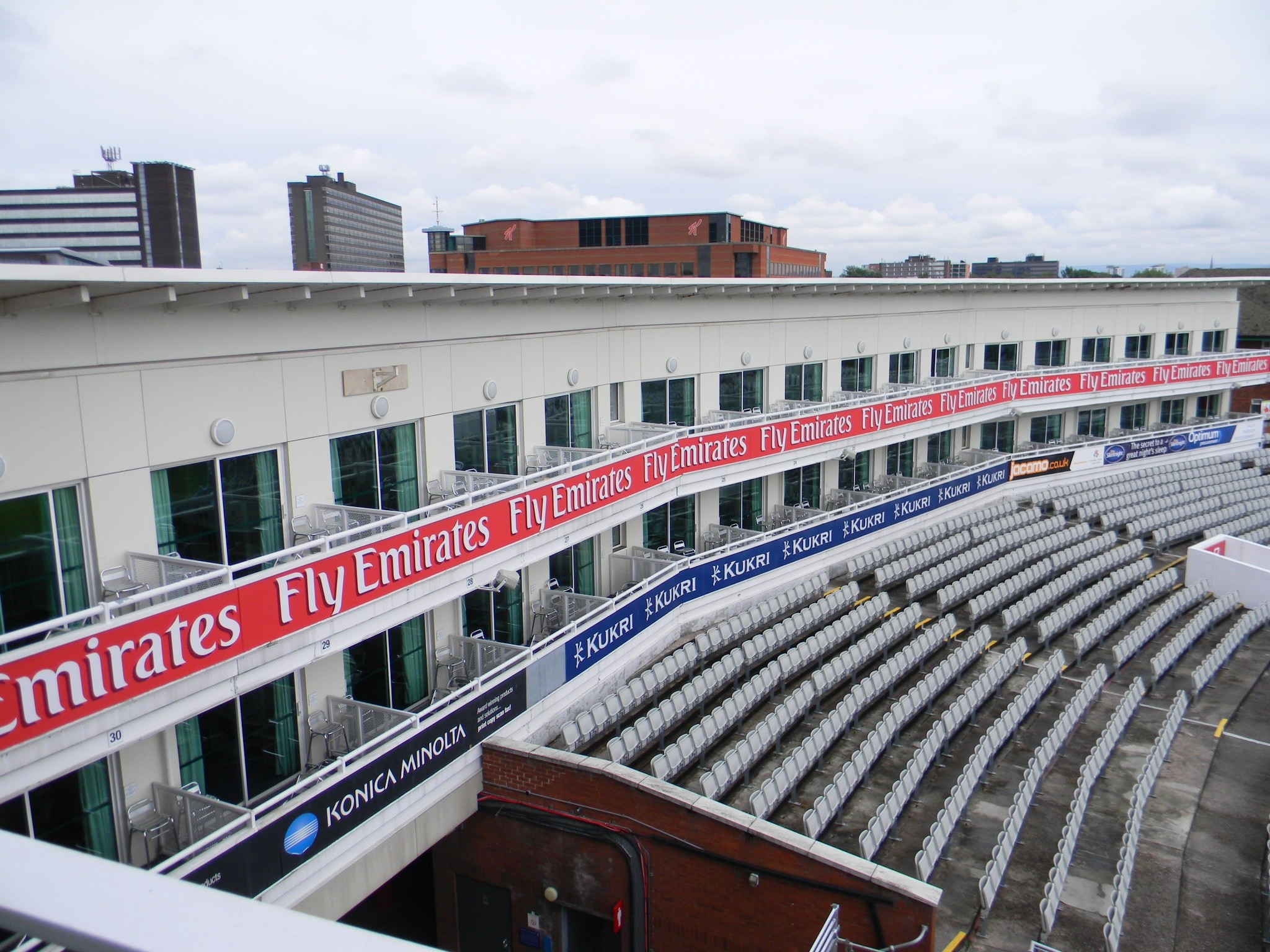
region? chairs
[305,709,350,766]
[530,577,578,637]
[127,798,180,868]
[433,630,502,690]
[163,551,210,593]
[175,781,221,840]
[290,451,562,551]
[559,446,1270,952]
[337,696,376,749]
[101,565,153,618]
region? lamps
[667,357,679,373]
[831,448,856,462]
[902,320,1224,349]
[484,380,497,400]
[857,340,866,353]
[741,351,751,365]
[371,395,389,419]
[544,886,559,902]
[1229,382,1242,390]
[475,569,519,593]
[211,418,235,446]
[1002,407,1024,417]
[568,368,579,386]
[804,346,813,359]
